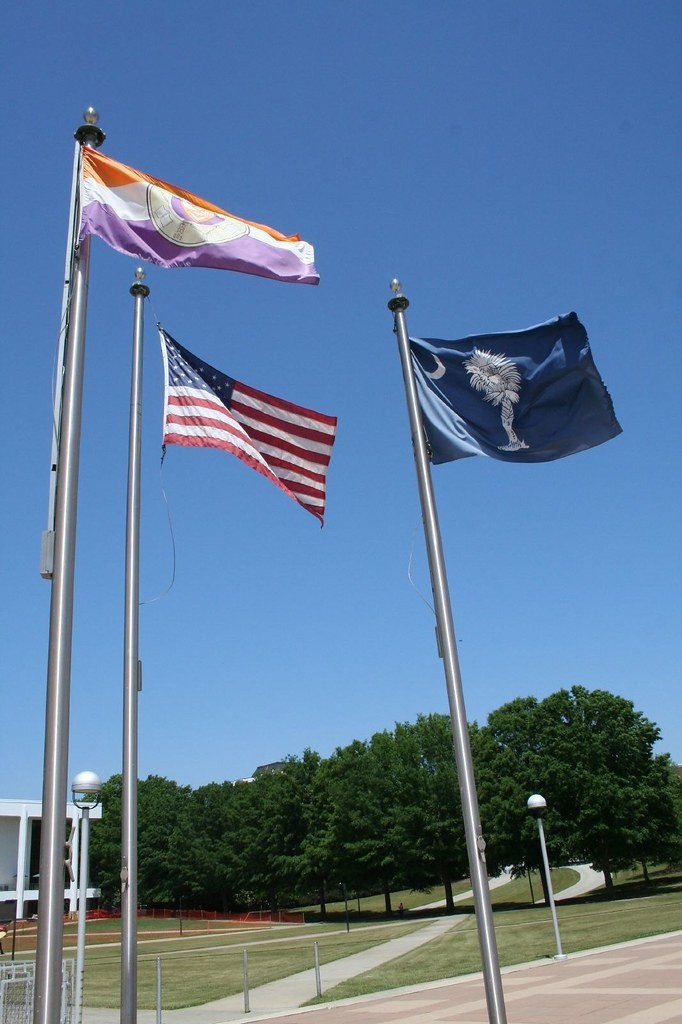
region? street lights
[68,771,102,1024]
[526,793,568,958]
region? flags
[75,144,320,285]
[405,312,623,465]
[157,328,339,528]
